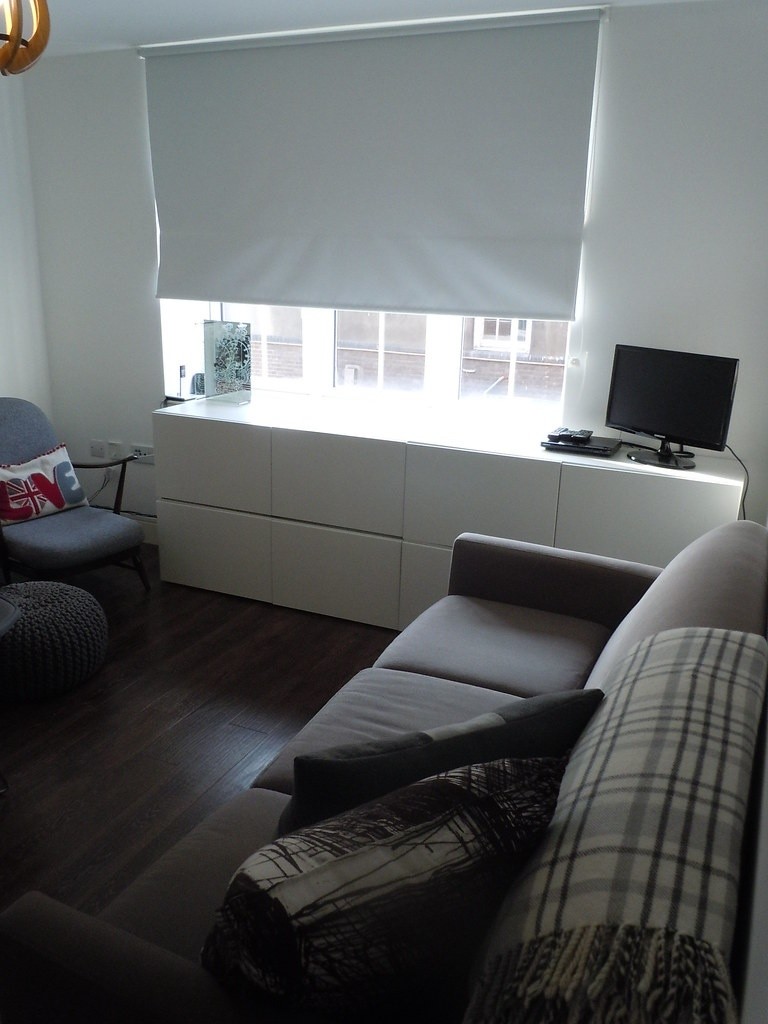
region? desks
[152,389,744,632]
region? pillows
[0,442,89,527]
[278,685,604,835]
[200,753,572,1016]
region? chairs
[0,398,152,594]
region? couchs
[1,516,767,1023]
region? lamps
[0,0,50,75]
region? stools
[1,581,110,700]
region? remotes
[549,427,593,442]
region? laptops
[540,435,623,457]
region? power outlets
[89,439,102,457]
[103,464,123,481]
[131,445,152,465]
[107,441,121,459]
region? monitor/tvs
[605,344,741,469]
